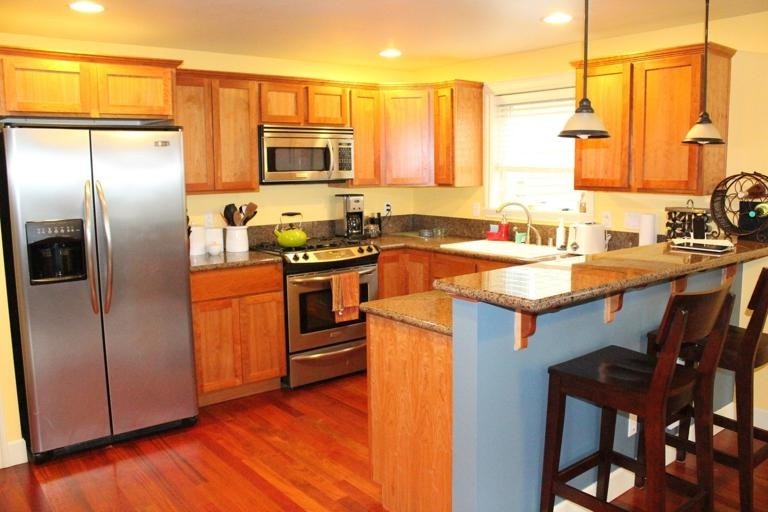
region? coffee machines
[334,193,364,242]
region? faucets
[496,201,530,244]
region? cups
[191,252,251,266]
[225,226,249,253]
[190,226,206,256]
[367,213,381,237]
[363,225,380,239]
[206,226,224,254]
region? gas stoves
[252,234,379,269]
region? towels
[330,271,360,324]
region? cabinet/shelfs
[175,79,481,193]
[365,314,453,512]
[407,249,517,294]
[189,263,288,395]
[574,42,731,195]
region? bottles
[748,201,768,221]
[737,183,765,200]
[578,192,586,212]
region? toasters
[566,221,609,256]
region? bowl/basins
[420,227,447,237]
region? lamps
[681,0,726,145]
[558,0,610,139]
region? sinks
[453,241,558,257]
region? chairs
[540,275,737,512]
[634,267,768,512]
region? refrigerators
[0,116,198,464]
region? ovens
[284,256,378,390]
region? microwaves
[259,124,355,184]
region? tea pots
[274,212,310,249]
